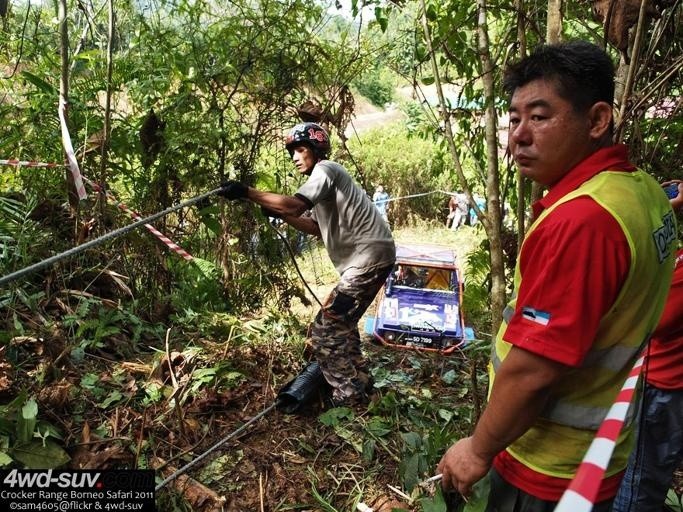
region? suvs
[362,244,474,357]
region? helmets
[285,122,330,151]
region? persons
[372,185,393,233]
[216,121,398,410]
[434,37,682,512]
[603,160,682,512]
[437,188,515,232]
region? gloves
[261,205,281,219]
[217,179,249,200]
